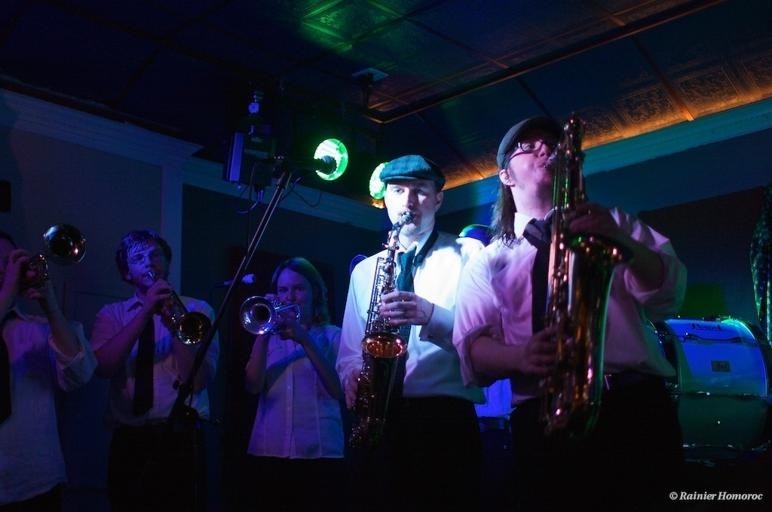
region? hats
[496,115,565,168]
[379,154,445,184]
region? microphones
[294,155,337,174]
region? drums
[652,316,772,469]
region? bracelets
[46,304,58,313]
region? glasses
[127,249,159,265]
[503,134,557,167]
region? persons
[0,230,99,512]
[458,224,512,433]
[240,257,345,462]
[450,113,689,512]
[333,154,487,512]
[90,230,221,511]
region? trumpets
[16,223,88,297]
[241,296,298,334]
[146,274,210,346]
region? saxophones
[540,114,625,448]
[350,213,414,448]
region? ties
[387,247,416,400]
[133,312,155,413]
[0,315,11,424]
